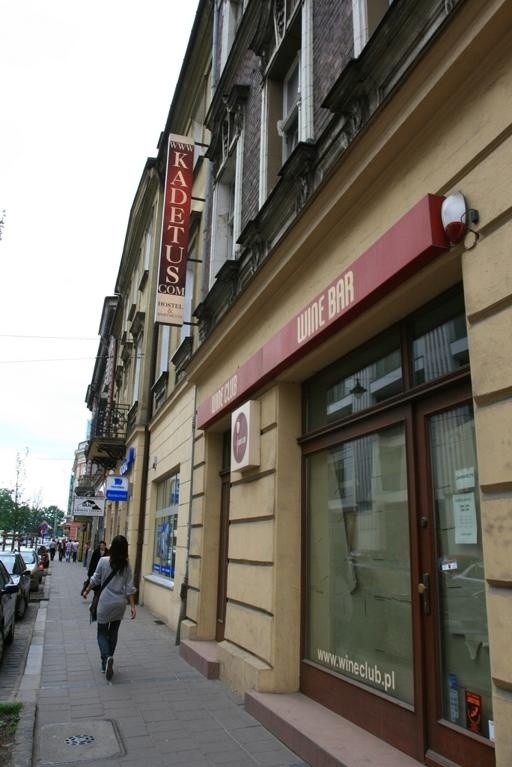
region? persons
[87,541,108,596]
[50,539,79,563]
[83,536,136,680]
[83,543,89,567]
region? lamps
[350,378,367,399]
[440,191,467,244]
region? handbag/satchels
[89,587,101,623]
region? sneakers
[101,658,113,681]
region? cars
[0,545,50,662]
